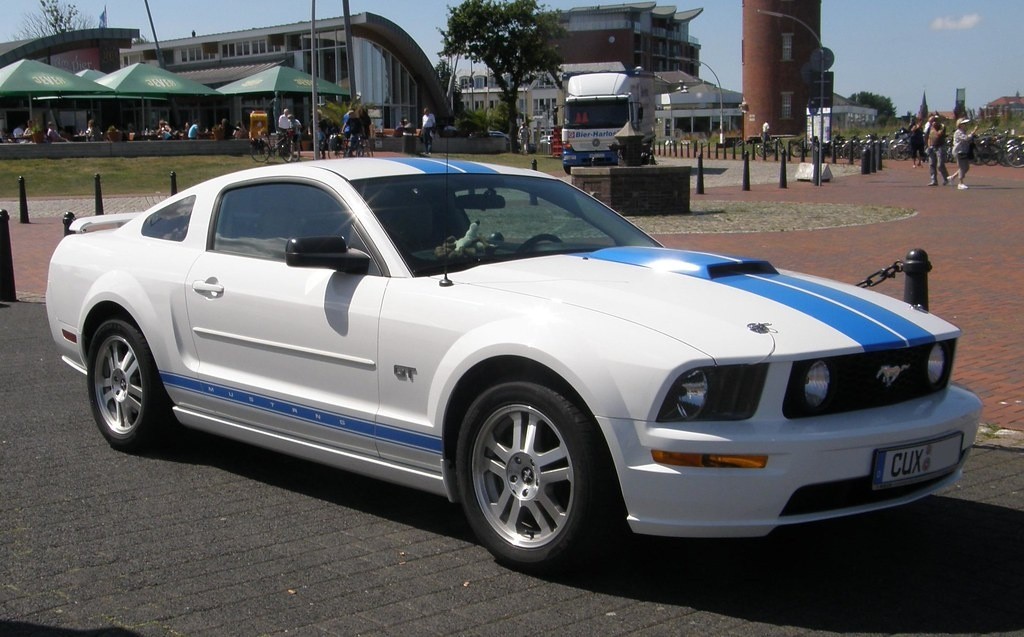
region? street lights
[674,56,725,144]
[756,8,824,187]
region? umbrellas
[0,60,349,133]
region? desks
[0,137,29,142]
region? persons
[947,117,979,189]
[906,115,951,186]
[519,123,530,154]
[0,104,373,156]
[762,120,770,144]
[408,201,451,248]
[395,108,436,156]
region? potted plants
[31,121,45,144]
[300,132,313,152]
[210,123,225,140]
[107,125,122,142]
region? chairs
[395,194,433,253]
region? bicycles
[756,136,786,158]
[889,126,1024,170]
[329,130,374,158]
[249,131,301,162]
[790,137,812,158]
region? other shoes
[917,163,923,167]
[943,179,947,185]
[927,181,938,186]
[913,165,915,168]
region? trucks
[559,67,657,174]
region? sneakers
[958,184,969,190]
[947,176,956,185]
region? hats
[957,119,970,126]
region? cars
[45,158,983,575]
[488,131,521,149]
[539,136,554,144]
